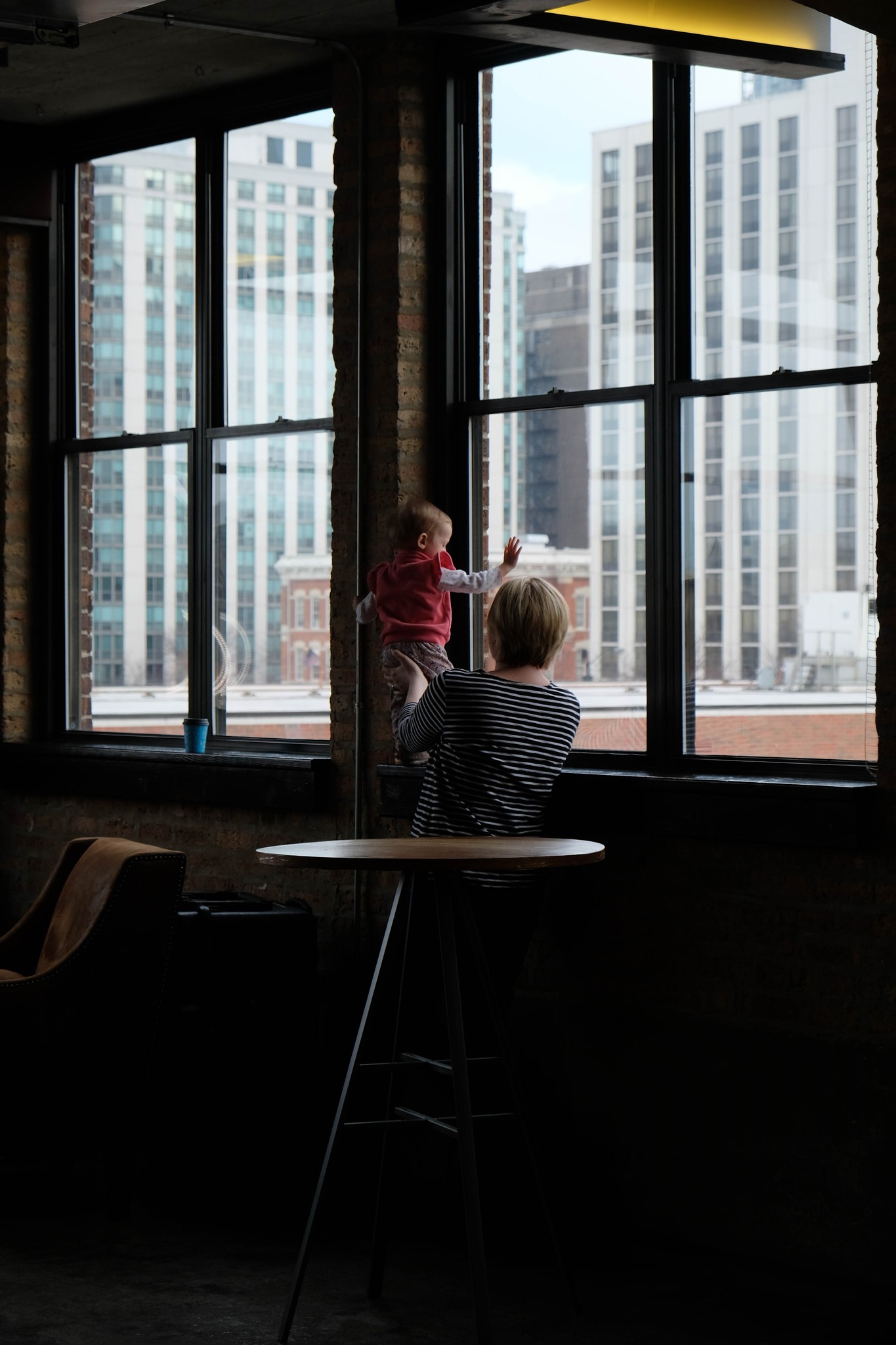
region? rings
[392,683,396,691]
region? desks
[258,837,609,1345]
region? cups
[182,718,209,754]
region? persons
[351,498,522,766]
[390,578,582,1177]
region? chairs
[0,836,188,1089]
